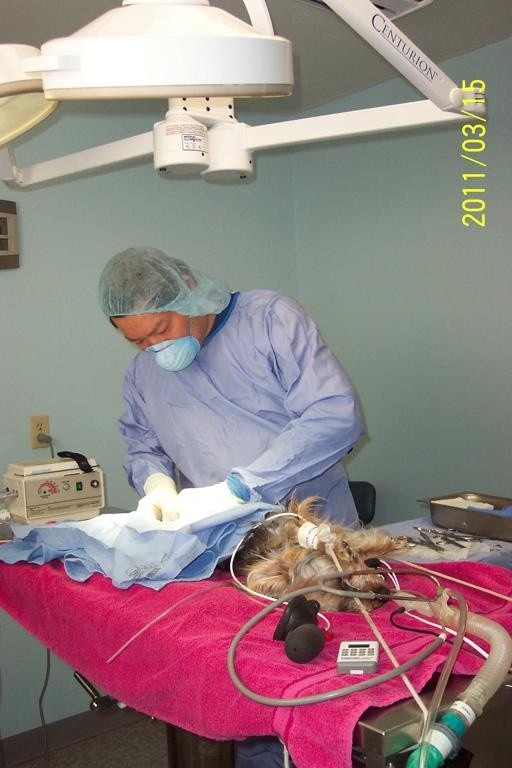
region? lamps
[42,2,297,101]
[0,43,62,149]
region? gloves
[163,482,232,522]
[139,473,178,520]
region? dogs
[215,495,410,612]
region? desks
[1,505,512,767]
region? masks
[145,336,200,371]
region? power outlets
[29,414,49,449]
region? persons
[99,246,367,768]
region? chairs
[348,481,377,525]
